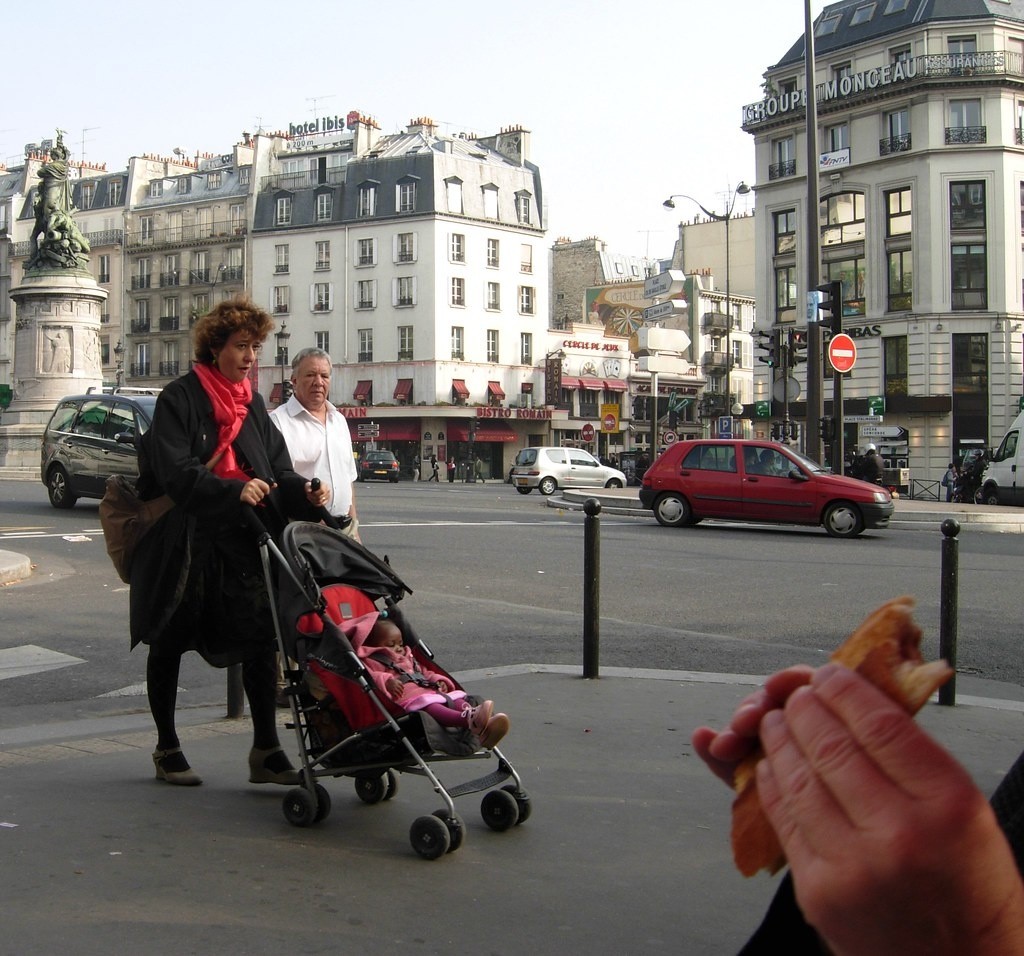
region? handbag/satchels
[99,474,154,584]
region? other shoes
[480,713,510,750]
[275,681,302,709]
[461,699,493,735]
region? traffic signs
[863,426,904,437]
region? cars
[639,439,895,540]
[357,450,399,483]
[511,446,628,495]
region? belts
[332,516,352,530]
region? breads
[730,597,956,878]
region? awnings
[269,385,284,403]
[447,419,518,443]
[344,418,421,441]
[353,381,372,400]
[487,382,505,400]
[561,376,628,393]
[393,379,413,399]
[453,380,470,399]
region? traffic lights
[789,327,807,367]
[771,421,801,440]
[818,416,832,443]
[474,416,480,432]
[759,329,781,369]
[816,280,842,334]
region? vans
[980,409,1024,507]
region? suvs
[40,386,164,509]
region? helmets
[864,443,876,453]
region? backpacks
[942,471,952,487]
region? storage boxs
[883,468,910,485]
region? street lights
[662,180,751,470]
[114,340,127,387]
[276,320,291,404]
[170,263,227,308]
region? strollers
[244,477,532,862]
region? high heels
[152,744,204,786]
[248,746,318,791]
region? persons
[861,443,885,485]
[265,345,362,707]
[412,454,486,484]
[756,450,780,477]
[129,297,330,787]
[942,449,986,503]
[691,661,1024,956]
[355,617,509,750]
[22,135,73,264]
[600,451,662,487]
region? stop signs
[603,414,616,430]
[828,334,857,372]
[582,424,594,441]
